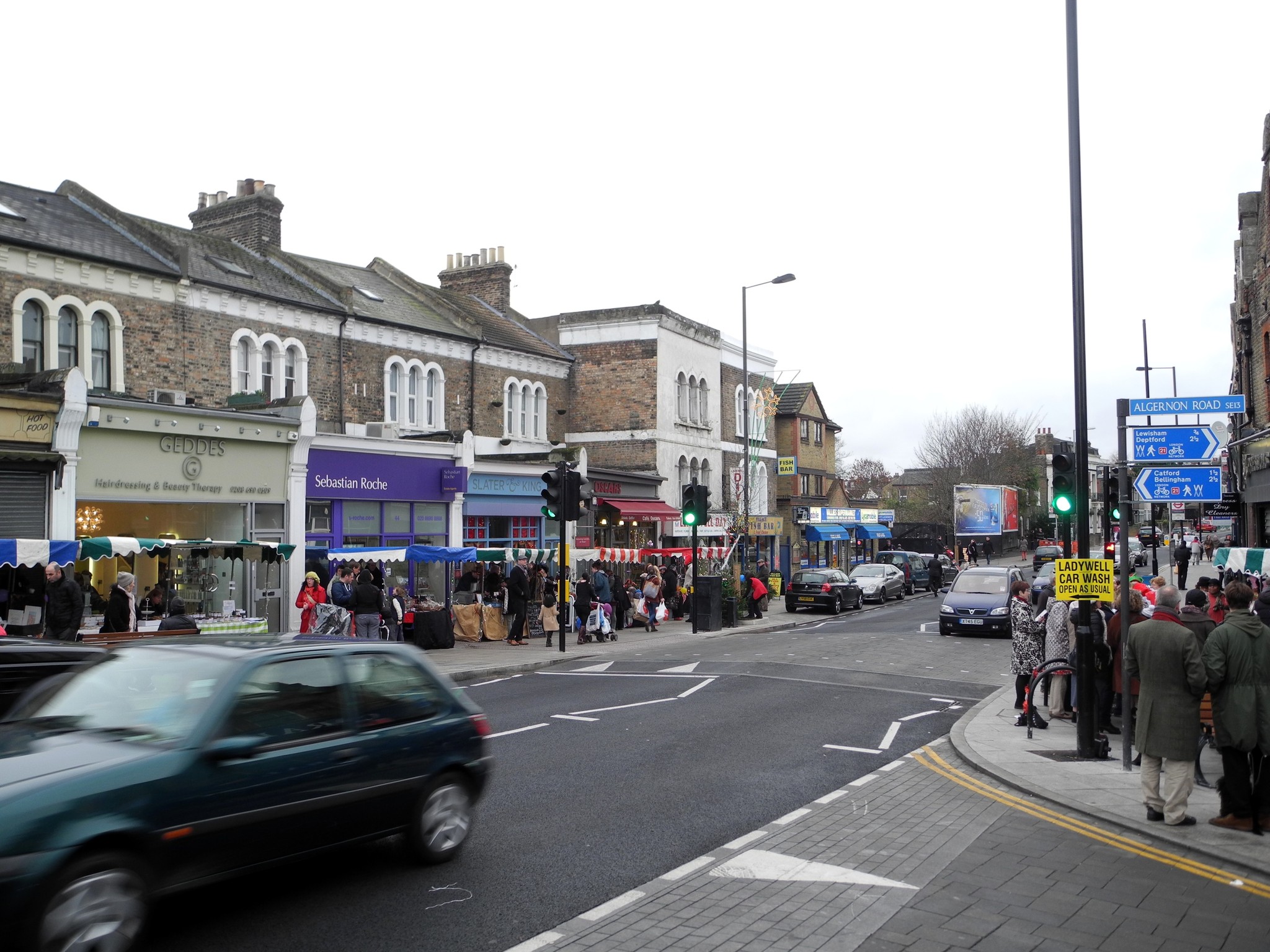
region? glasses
[1024,588,1031,592]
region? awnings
[805,524,850,542]
[597,496,682,522]
[1212,546,1270,577]
[0,449,67,490]
[855,523,893,539]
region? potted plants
[492,389,504,407]
[557,401,567,415]
[499,426,511,446]
[227,390,269,405]
[550,432,560,446]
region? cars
[0,633,495,952]
[1196,520,1217,533]
[847,563,905,605]
[1031,562,1055,604]
[784,568,864,614]
[1170,526,1195,538]
[1072,541,1149,574]
[919,553,959,588]
[1033,545,1066,571]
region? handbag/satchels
[642,577,660,599]
[1067,647,1100,673]
[586,603,611,634]
[637,598,650,618]
[1205,545,1210,549]
[1014,704,1048,729]
[655,600,668,624]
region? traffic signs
[1133,466,1222,503]
[1131,426,1220,461]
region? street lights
[1137,365,1181,425]
[745,272,797,574]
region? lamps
[76,506,105,533]
[107,414,129,424]
[155,419,177,427]
[240,427,261,435]
[277,430,297,438]
[199,423,220,432]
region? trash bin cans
[697,576,722,632]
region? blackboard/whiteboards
[526,600,546,638]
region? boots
[645,621,658,632]
[577,625,588,644]
[546,637,552,647]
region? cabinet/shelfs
[165,557,215,614]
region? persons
[0,559,769,649]
[1008,531,1270,837]
[883,540,903,551]
[928,533,995,597]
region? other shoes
[1015,700,1121,735]
[745,615,763,618]
[760,609,768,612]
[685,619,692,622]
[1179,587,1187,590]
[1146,807,1269,833]
[507,639,528,645]
[674,617,683,620]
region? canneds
[230,611,242,621]
[187,611,224,624]
[162,612,168,619]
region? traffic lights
[1104,542,1115,562]
[541,470,589,521]
[1103,476,1133,522]
[1052,452,1076,514]
[681,484,712,526]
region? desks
[404,610,455,649]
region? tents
[640,547,731,575]
[569,547,642,575]
[0,538,80,568]
[325,544,477,609]
[475,547,559,563]
[76,537,297,620]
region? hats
[1197,576,1211,587]
[658,565,667,570]
[517,555,528,560]
[1185,589,1208,607]
[471,566,481,575]
[170,596,186,612]
[305,571,320,583]
[757,559,764,563]
[1207,578,1220,587]
[115,571,134,588]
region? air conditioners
[148,389,186,406]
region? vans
[875,551,931,595]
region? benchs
[1131,693,1216,789]
[75,628,202,645]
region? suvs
[939,563,1028,639]
[1135,526,1166,547]
